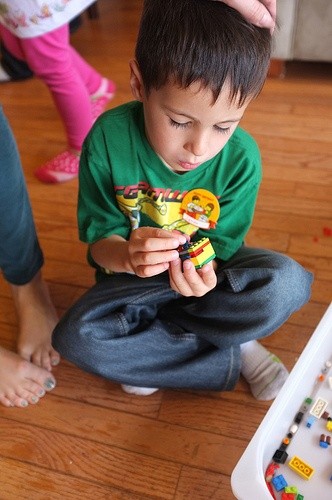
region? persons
[50,0,314,401]
[0,0,276,407]
[0,0,117,185]
